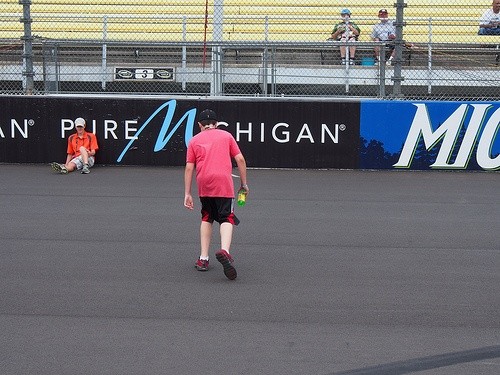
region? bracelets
[240,184,247,187]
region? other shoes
[374,58,379,66]
[350,58,354,65]
[340,57,345,65]
[386,59,391,65]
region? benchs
[1,0,500,44]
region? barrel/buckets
[359,57,376,66]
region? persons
[51,118,98,174]
[370,9,396,66]
[478,0,500,48]
[184,110,250,279]
[331,9,361,65]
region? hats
[196,109,218,125]
[75,118,85,127]
[341,9,351,16]
[379,9,388,15]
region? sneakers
[215,249,237,280]
[195,257,209,271]
[81,164,90,174]
[51,162,67,174]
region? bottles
[238,189,246,206]
